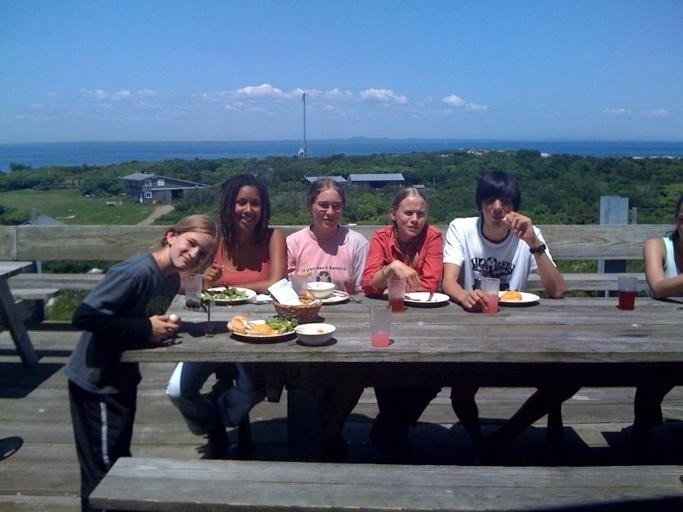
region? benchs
[88,457,682,512]
[9,286,61,325]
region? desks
[0,260,46,371]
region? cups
[618,275,638,310]
[480,277,499,312]
[368,305,391,347]
[387,273,405,312]
[183,273,202,309]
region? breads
[501,291,522,301]
[226,316,271,335]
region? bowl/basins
[293,321,336,345]
[307,282,336,298]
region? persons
[167,175,287,461]
[284,177,370,460]
[63,212,220,511]
[360,185,444,464]
[440,169,584,467]
[631,196,682,464]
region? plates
[201,286,256,303]
[227,319,296,339]
[499,290,541,304]
[404,292,449,304]
[322,290,350,304]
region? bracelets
[528,242,546,254]
[380,265,387,278]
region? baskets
[272,295,322,324]
